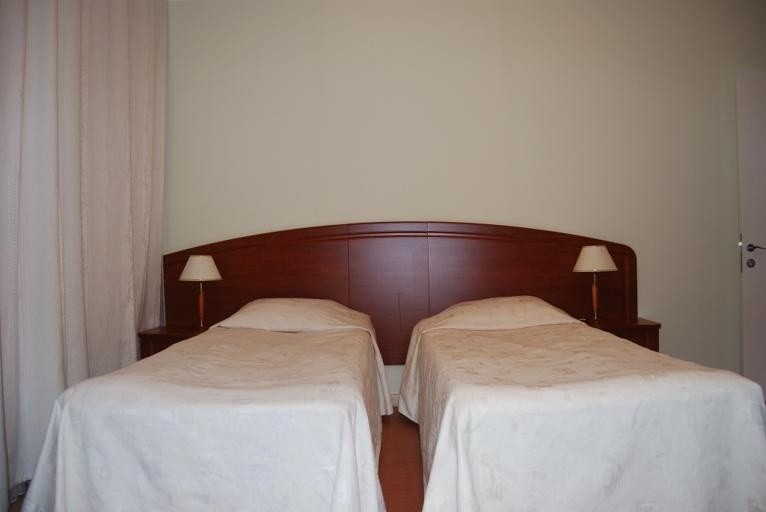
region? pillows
[437,293,578,334]
[214,296,366,330]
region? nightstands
[136,324,203,360]
[584,315,664,352]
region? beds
[17,218,394,512]
[394,219,766,511]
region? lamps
[178,249,224,327]
[572,244,622,320]
